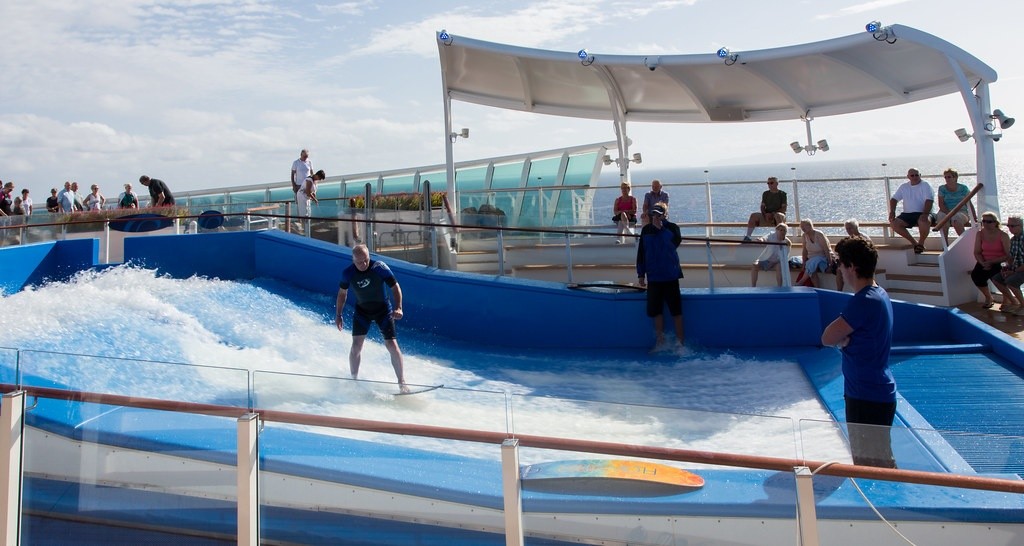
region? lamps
[601,153,615,165]
[644,55,662,72]
[991,108,1016,129]
[439,27,453,46]
[449,127,470,143]
[865,20,898,46]
[577,48,596,66]
[631,152,643,164]
[790,141,801,154]
[717,47,738,67]
[952,127,970,144]
[816,139,830,152]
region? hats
[650,205,666,214]
[91,184,99,189]
[52,189,58,193]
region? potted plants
[338,191,449,248]
[53,204,193,262]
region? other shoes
[741,236,751,243]
[615,240,621,243]
[1008,305,1024,316]
[914,245,925,253]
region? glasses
[1007,224,1020,228]
[651,211,662,217]
[981,219,996,224]
[836,259,841,267]
[945,176,953,179]
[7,187,14,192]
[909,174,920,177]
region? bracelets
[337,315,342,316]
[54,207,55,210]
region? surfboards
[384,384,444,397]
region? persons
[139,175,175,207]
[832,218,873,291]
[888,168,934,254]
[83,184,105,211]
[937,169,978,250]
[334,245,409,393]
[971,212,1024,315]
[795,218,833,287]
[46,182,83,238]
[118,184,139,208]
[741,177,787,244]
[0,180,32,246]
[822,237,898,469]
[641,180,669,226]
[291,149,325,235]
[751,223,792,288]
[637,202,685,345]
[611,182,637,244]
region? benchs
[459,213,971,237]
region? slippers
[998,303,1010,310]
[983,301,994,309]
